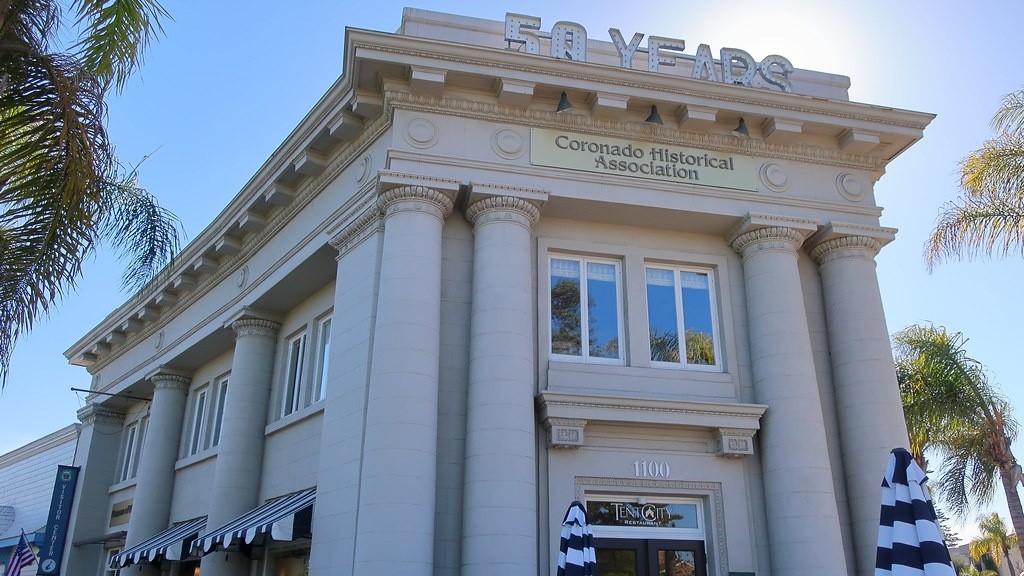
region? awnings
[188,483,317,557]
[106,515,208,571]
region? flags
[3,531,35,576]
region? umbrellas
[873,447,957,575]
[556,500,597,576]
